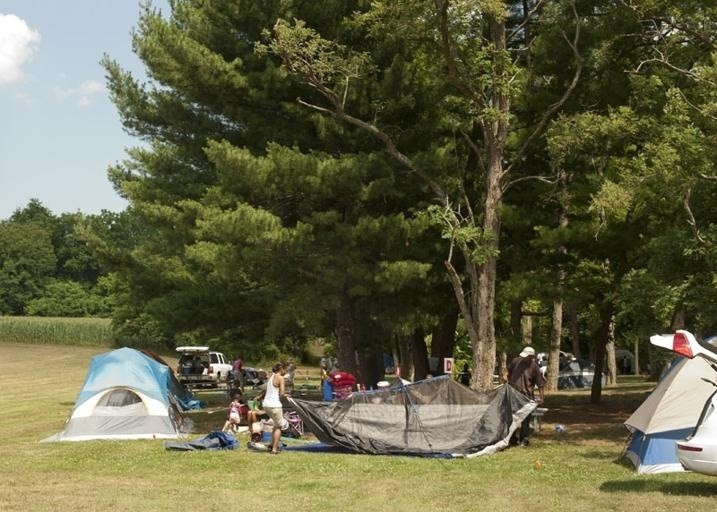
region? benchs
[184,381,233,400]
[531,408,549,433]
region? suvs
[173,345,233,390]
[643,325,716,479]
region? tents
[41,345,205,442]
[616,336,717,476]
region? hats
[518,346,534,358]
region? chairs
[287,411,304,436]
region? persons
[227,356,339,453]
[538,365,547,401]
[456,363,472,387]
[504,347,547,446]
